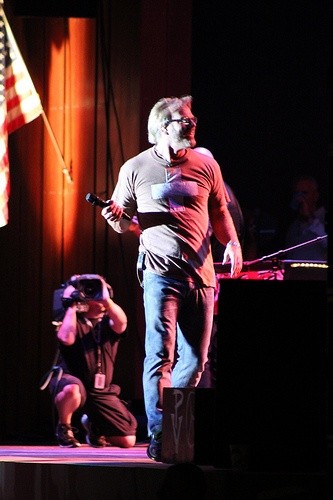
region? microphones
[85,192,131,221]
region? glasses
[168,116,198,124]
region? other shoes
[81,414,106,448]
[56,424,80,448]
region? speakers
[219,279,333,474]
[161,387,222,467]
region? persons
[101,97,243,459]
[282,176,328,260]
[49,274,137,447]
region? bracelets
[228,241,240,248]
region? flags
[0,0,43,228]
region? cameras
[61,279,104,309]
